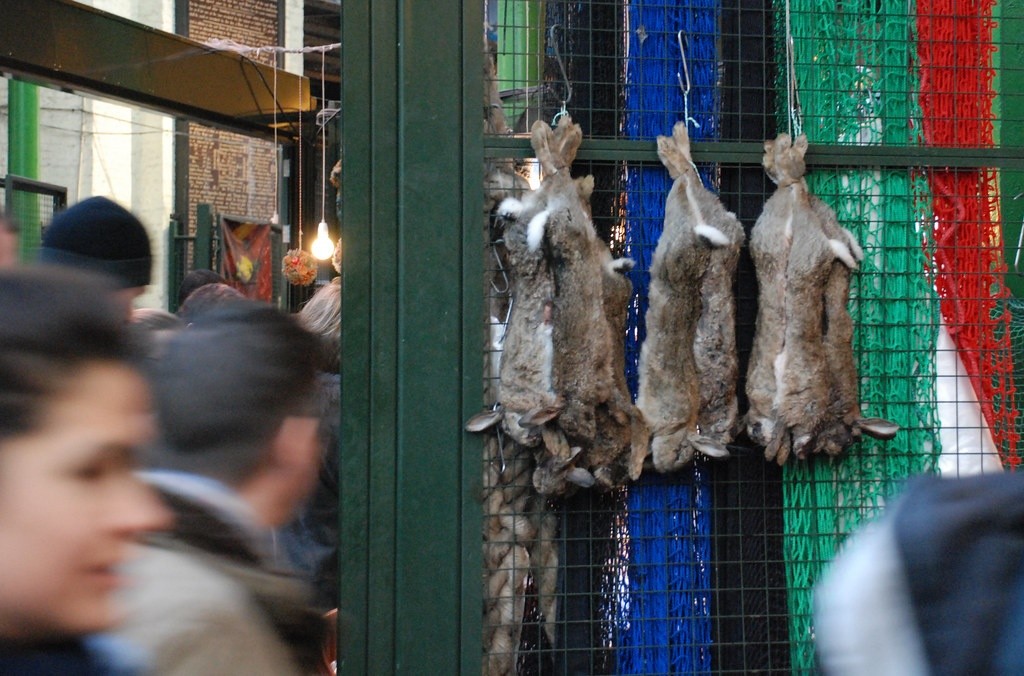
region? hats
[40,196,152,290]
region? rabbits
[463,114,901,500]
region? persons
[127,269,340,608]
[1,262,181,675]
[39,194,152,330]
[0,216,23,281]
[111,294,339,676]
